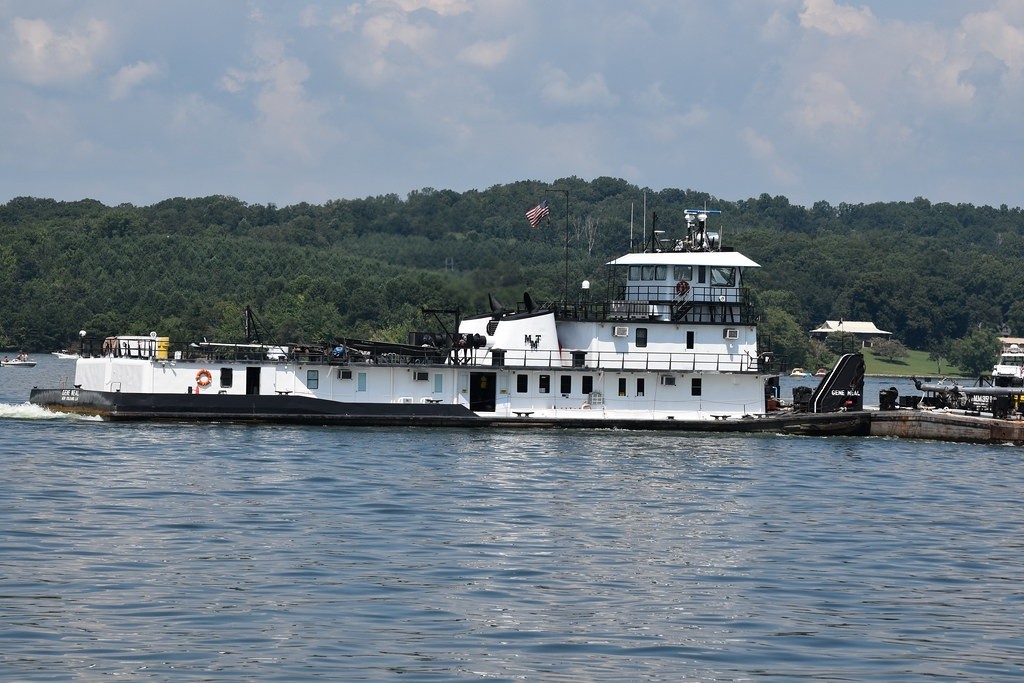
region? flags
[525,200,550,229]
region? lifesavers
[196,370,211,386]
[676,281,690,295]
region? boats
[790,368,807,378]
[51,352,81,359]
[815,368,827,377]
[992,338,1024,389]
[0,360,38,368]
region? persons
[3,352,28,362]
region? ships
[28,206,873,438]
